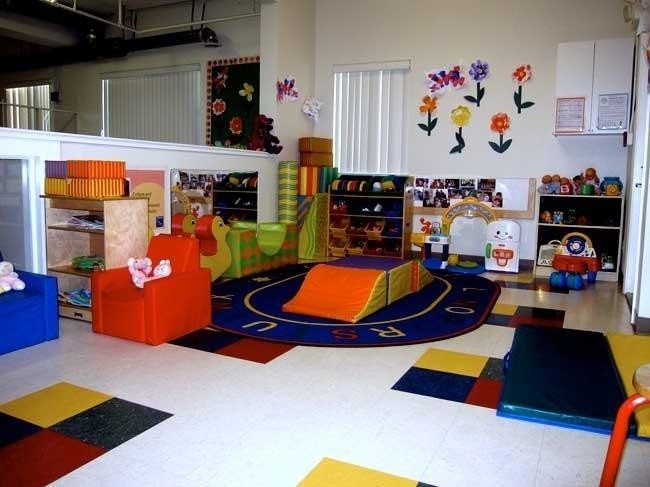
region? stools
[599,363,650,487]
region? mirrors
[170,169,260,237]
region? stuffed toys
[248,114,283,154]
[0,261,26,293]
[142,259,172,282]
[127,257,152,288]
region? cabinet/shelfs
[533,194,625,283]
[39,195,149,323]
[326,177,414,261]
[554,37,637,138]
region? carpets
[208,262,501,347]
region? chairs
[91,236,211,346]
[0,250,59,356]
